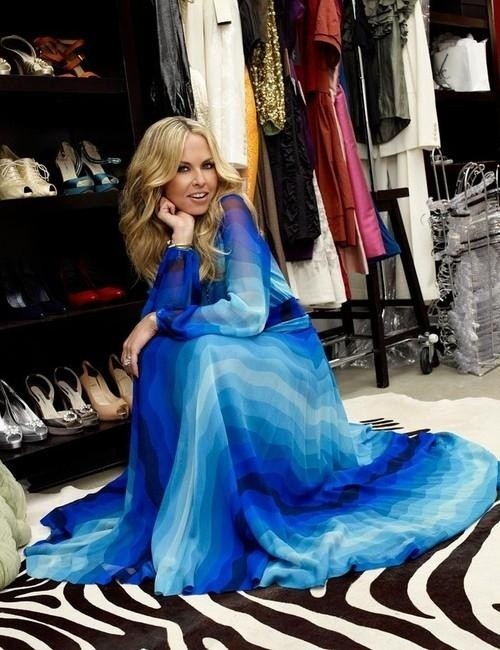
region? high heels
[0,354,133,451]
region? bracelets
[166,240,192,248]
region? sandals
[1,139,122,200]
[0,34,100,77]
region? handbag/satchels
[433,34,491,92]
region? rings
[124,356,131,368]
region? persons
[24,118,500,597]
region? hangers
[420,161,500,377]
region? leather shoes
[0,284,125,320]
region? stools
[306,187,430,388]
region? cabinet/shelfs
[0,37,129,494]
